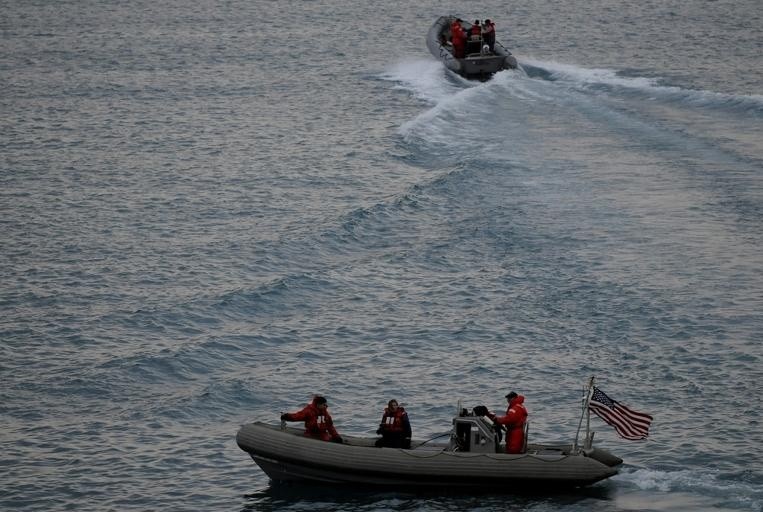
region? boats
[236,421,623,494]
[426,15,517,81]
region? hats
[505,391,517,399]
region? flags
[590,386,654,441]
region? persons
[482,19,496,52]
[281,394,343,444]
[492,392,528,454]
[467,20,483,38]
[451,17,467,60]
[375,399,411,448]
